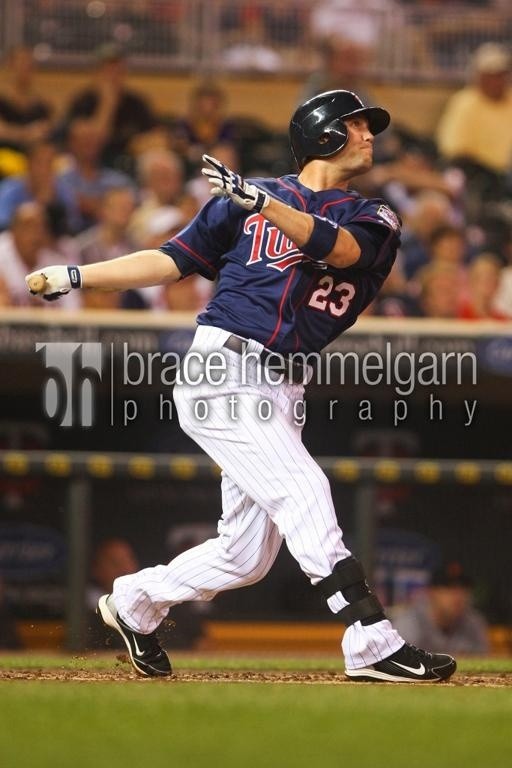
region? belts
[223,335,308,384]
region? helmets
[290,90,391,169]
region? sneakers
[95,591,172,677]
[343,642,457,682]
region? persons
[87,530,139,604]
[1,0,512,321]
[25,91,457,682]
[395,557,493,656]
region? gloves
[201,153,270,214]
[25,264,83,301]
